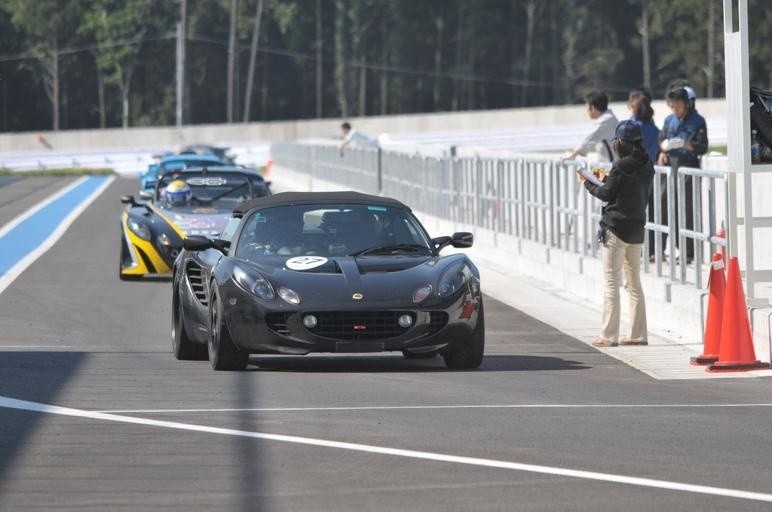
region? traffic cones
[690,253,769,372]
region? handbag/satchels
[597,228,607,242]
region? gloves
[660,137,684,152]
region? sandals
[592,336,648,348]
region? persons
[614,84,661,262]
[563,87,618,251]
[659,88,706,266]
[336,122,382,156]
[665,81,692,89]
[578,120,655,347]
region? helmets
[266,212,304,246]
[164,180,193,206]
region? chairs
[269,210,304,238]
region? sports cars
[171,192,484,370]
[119,143,274,281]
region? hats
[610,120,643,142]
[684,87,696,100]
